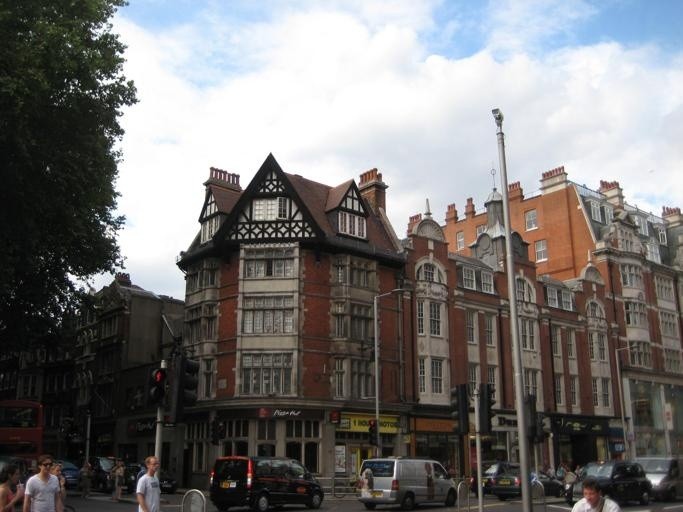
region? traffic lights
[183,357,200,407]
[369,420,377,433]
[449,383,469,435]
[480,383,496,433]
[148,368,167,401]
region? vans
[356,458,460,512]
[210,456,324,511]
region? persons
[569,480,623,512]
[0,454,162,512]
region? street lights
[614,345,639,459]
[492,107,534,512]
[373,288,405,457]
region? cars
[59,456,178,494]
[0,455,29,493]
[469,456,683,507]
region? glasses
[40,462,53,467]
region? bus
[1,400,44,474]
